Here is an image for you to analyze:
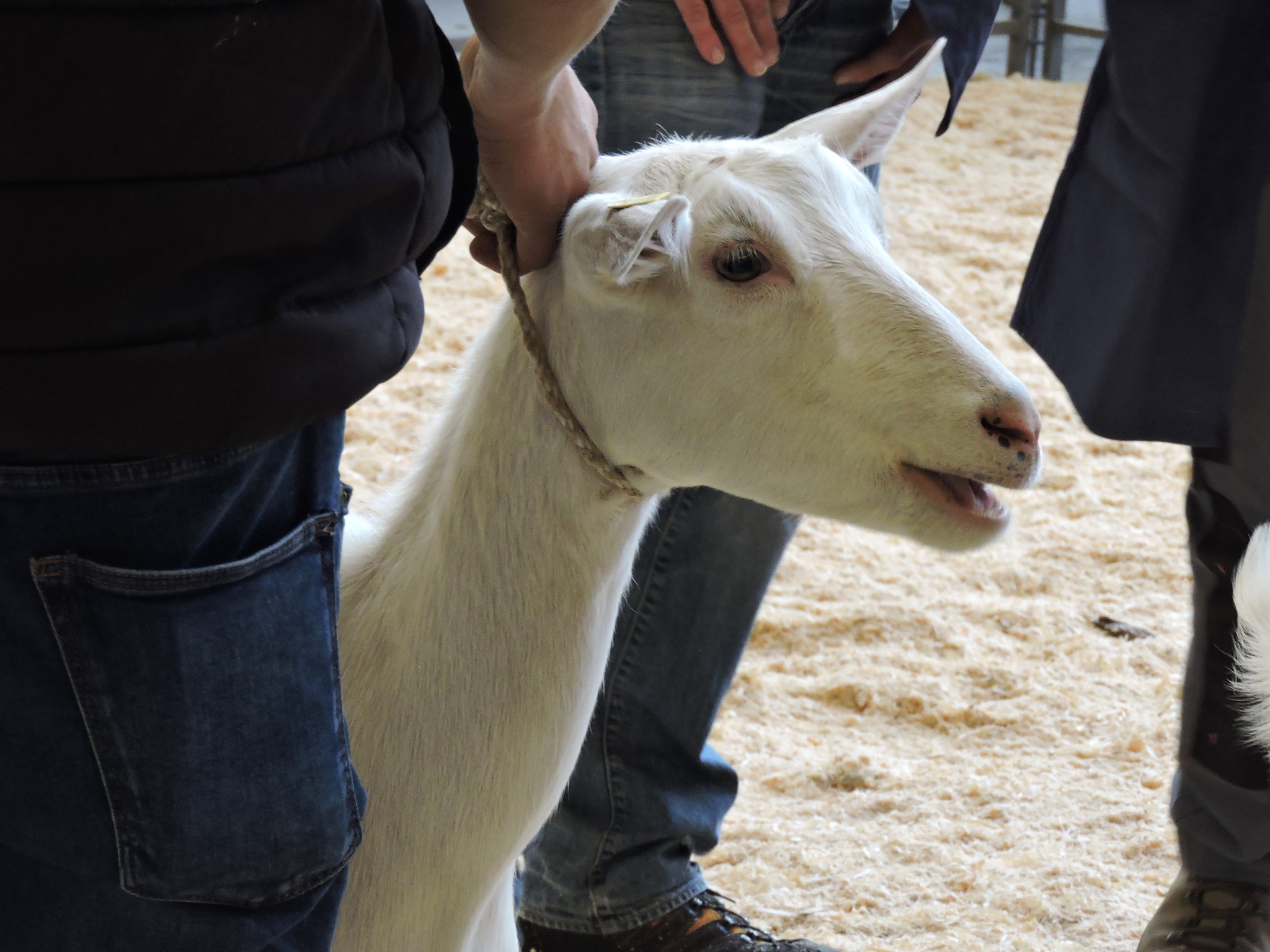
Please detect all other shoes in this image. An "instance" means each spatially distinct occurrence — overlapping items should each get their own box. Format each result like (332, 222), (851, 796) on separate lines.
(516, 889), (836, 952)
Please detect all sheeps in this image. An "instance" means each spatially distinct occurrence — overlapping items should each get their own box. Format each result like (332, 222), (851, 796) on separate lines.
(1227, 522), (1270, 756)
(325, 35), (1048, 952)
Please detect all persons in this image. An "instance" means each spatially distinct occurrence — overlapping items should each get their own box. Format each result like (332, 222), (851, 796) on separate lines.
(0, 0), (623, 952)
(501, 0), (1013, 952)
(1010, 0), (1270, 952)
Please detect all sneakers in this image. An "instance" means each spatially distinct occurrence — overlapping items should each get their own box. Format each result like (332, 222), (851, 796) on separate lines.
(1138, 869), (1269, 952)
